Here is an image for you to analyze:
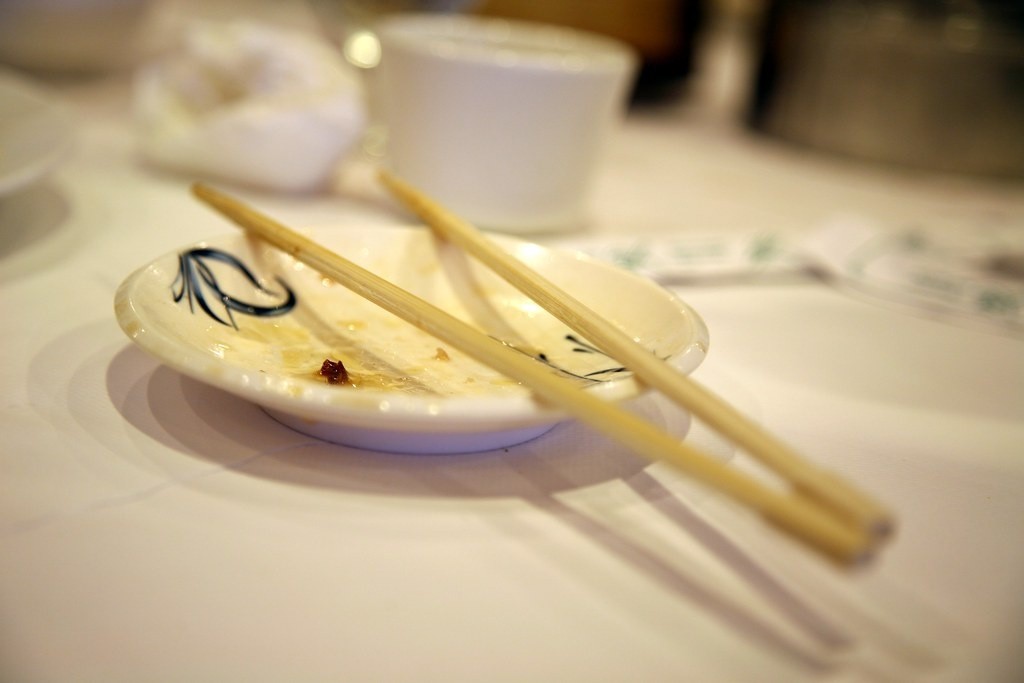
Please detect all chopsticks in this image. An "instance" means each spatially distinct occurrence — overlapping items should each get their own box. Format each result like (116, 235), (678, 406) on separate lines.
(193, 170), (893, 566)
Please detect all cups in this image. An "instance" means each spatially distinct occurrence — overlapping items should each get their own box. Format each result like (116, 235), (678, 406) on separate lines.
(384, 14), (632, 235)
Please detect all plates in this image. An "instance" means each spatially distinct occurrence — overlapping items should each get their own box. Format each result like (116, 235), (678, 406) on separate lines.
(0, 81), (77, 193)
(115, 228), (708, 453)
